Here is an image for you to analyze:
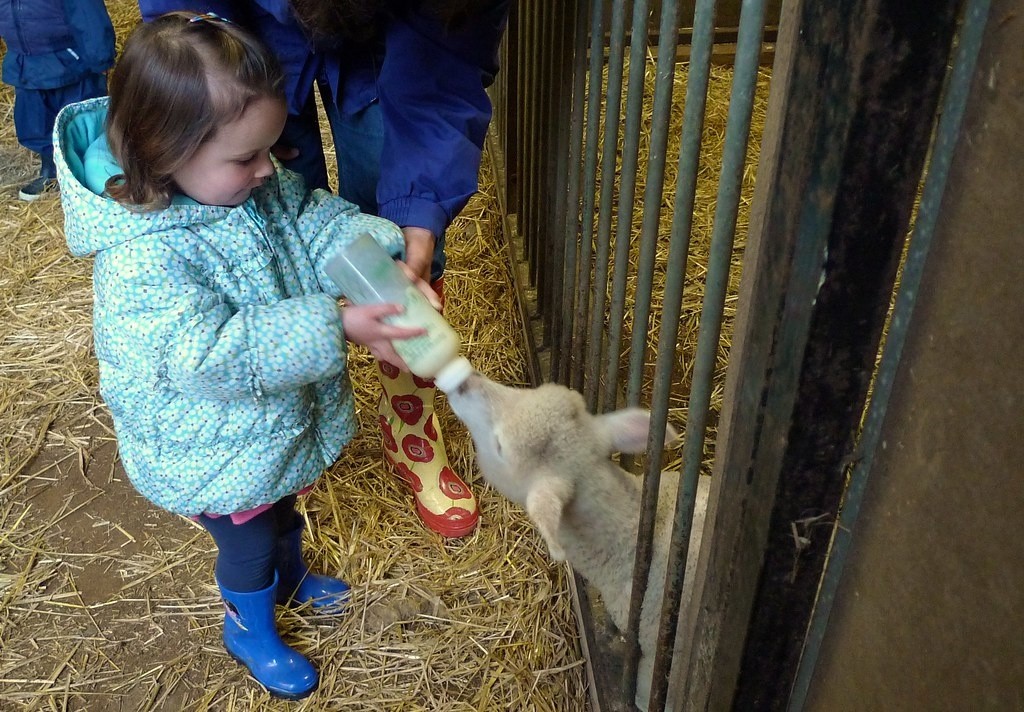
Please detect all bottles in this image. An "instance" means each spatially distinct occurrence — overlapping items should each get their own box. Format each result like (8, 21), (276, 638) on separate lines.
(322, 232), (473, 393)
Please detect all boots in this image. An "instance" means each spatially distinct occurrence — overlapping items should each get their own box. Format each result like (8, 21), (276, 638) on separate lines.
(373, 358), (480, 539)
(216, 564), (319, 699)
(269, 511), (351, 613)
(18, 145), (59, 200)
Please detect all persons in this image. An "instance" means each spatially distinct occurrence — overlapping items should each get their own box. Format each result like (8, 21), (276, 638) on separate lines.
(0, 0), (118, 202)
(137, 0), (512, 537)
(53, 9), (445, 700)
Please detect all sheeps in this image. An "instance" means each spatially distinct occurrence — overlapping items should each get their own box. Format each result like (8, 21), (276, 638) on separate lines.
(438, 372), (712, 712)
